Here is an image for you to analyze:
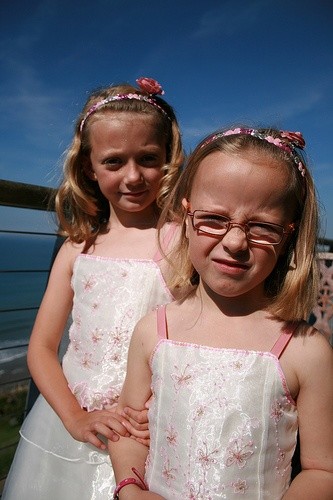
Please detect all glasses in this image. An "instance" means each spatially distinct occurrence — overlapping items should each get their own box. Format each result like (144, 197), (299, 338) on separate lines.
(186, 209), (297, 246)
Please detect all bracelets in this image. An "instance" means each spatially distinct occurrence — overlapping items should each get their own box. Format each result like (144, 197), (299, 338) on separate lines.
(112, 465), (150, 499)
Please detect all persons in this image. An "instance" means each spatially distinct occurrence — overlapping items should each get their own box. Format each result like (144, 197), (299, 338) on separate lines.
(106, 127), (333, 500)
(0, 76), (185, 500)
